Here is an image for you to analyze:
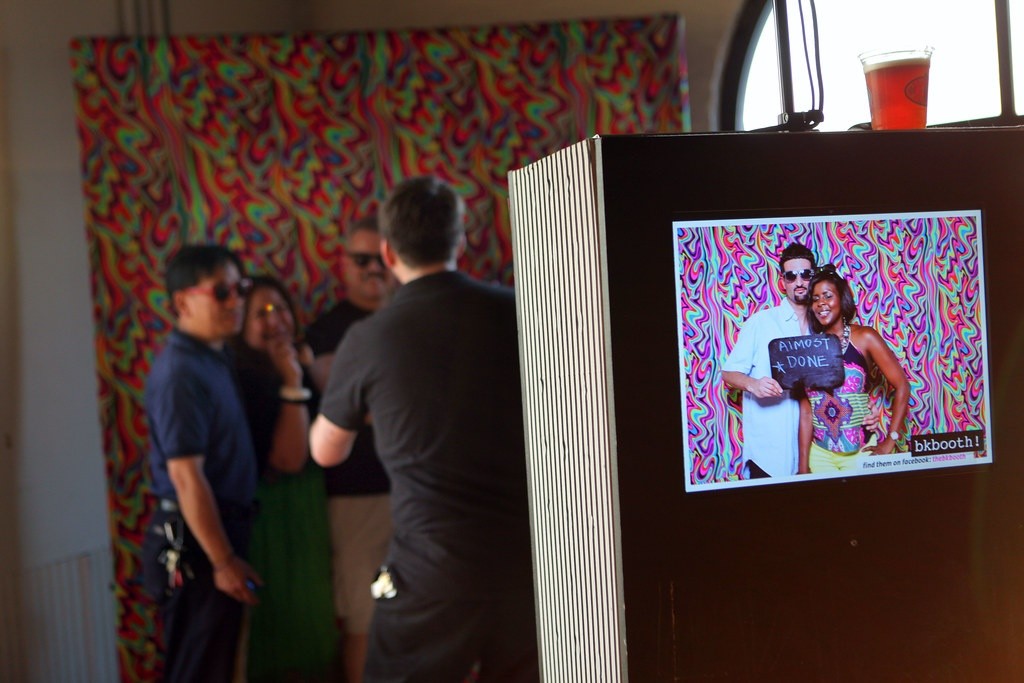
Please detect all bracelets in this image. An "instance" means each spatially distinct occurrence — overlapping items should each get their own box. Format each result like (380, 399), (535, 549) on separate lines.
(279, 387), (312, 400)
(216, 554), (236, 571)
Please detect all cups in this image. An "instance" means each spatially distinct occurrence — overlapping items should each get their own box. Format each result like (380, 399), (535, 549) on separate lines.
(859, 46), (932, 129)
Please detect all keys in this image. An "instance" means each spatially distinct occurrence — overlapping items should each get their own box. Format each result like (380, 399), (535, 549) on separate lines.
(165, 551), (182, 586)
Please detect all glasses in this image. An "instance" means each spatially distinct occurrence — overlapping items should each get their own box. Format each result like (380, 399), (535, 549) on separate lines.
(344, 250), (385, 270)
(185, 281), (243, 303)
(814, 264), (835, 275)
(781, 269), (814, 282)
(252, 303), (288, 316)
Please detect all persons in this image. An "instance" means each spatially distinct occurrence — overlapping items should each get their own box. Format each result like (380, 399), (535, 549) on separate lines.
(299, 204), (395, 683)
(797, 264), (911, 474)
(722, 244), (816, 479)
(307, 177), (538, 683)
(137, 244), (266, 683)
(229, 275), (343, 683)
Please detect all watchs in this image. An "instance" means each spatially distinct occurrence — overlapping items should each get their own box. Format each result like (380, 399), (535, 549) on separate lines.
(889, 431), (901, 441)
